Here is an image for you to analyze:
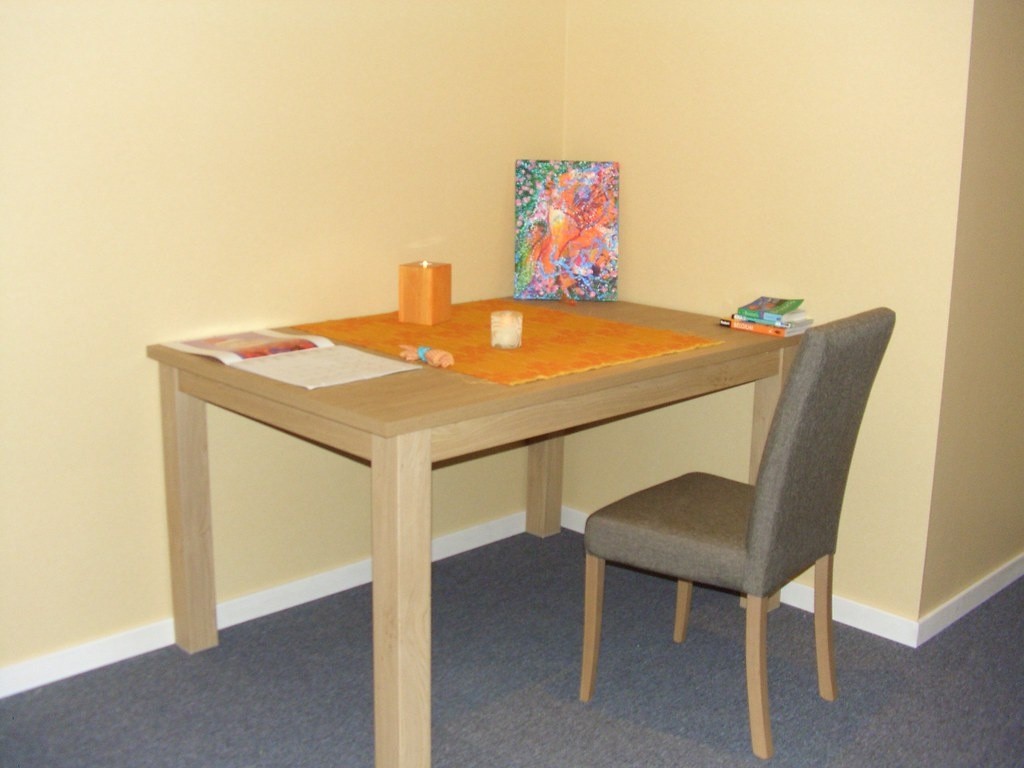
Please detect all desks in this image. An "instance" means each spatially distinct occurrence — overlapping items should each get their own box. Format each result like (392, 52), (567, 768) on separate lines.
(143, 294), (813, 768)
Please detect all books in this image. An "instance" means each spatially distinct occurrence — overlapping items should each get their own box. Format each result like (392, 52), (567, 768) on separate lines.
(720, 296), (814, 338)
(160, 329), (423, 390)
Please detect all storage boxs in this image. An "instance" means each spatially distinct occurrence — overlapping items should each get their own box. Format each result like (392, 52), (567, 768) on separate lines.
(397, 260), (452, 325)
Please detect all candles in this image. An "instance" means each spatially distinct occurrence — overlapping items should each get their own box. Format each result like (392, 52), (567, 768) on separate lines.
(489, 310), (523, 349)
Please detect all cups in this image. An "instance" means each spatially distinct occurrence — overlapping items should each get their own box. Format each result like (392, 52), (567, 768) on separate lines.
(491, 310), (523, 349)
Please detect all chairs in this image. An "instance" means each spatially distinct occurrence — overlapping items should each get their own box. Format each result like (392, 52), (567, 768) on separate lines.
(572, 298), (899, 760)
